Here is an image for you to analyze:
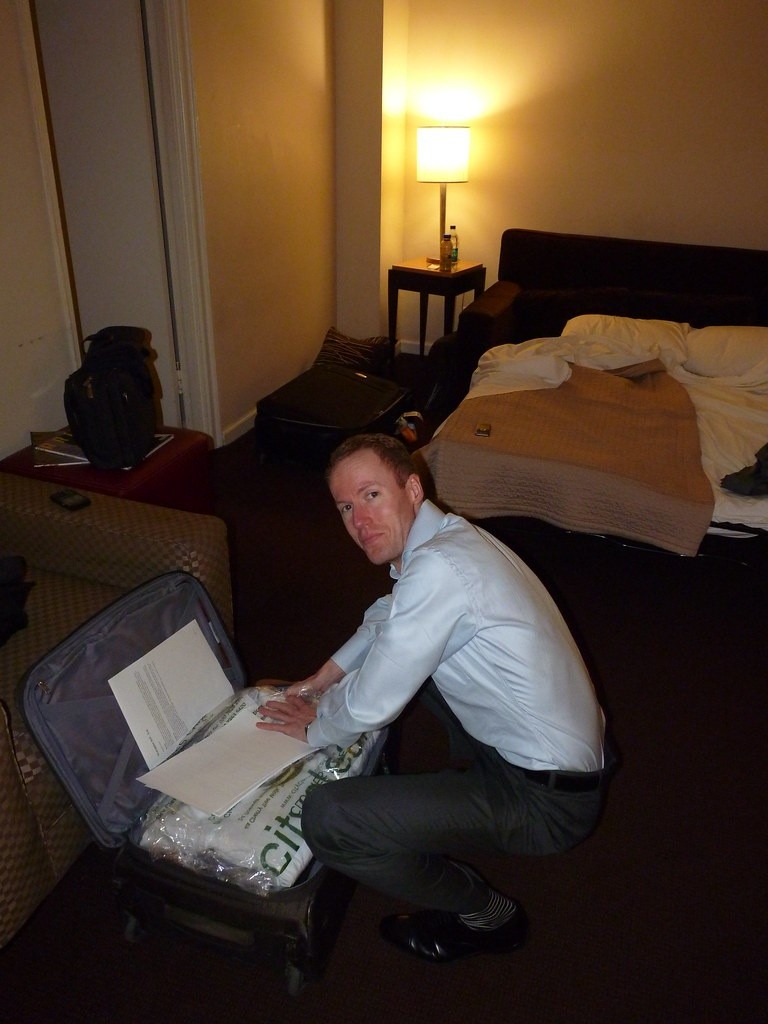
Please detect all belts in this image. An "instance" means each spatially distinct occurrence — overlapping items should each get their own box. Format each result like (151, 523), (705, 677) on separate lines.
(510, 765), (598, 793)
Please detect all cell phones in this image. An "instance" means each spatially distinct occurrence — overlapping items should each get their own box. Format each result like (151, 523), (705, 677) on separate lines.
(50, 489), (91, 511)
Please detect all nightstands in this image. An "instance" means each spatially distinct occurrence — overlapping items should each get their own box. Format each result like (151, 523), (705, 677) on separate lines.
(388, 267), (486, 355)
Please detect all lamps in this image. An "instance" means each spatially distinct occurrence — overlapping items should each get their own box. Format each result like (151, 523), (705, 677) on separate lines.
(392, 125), (484, 276)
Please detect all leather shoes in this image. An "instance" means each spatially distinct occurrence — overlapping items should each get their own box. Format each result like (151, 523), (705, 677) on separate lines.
(378, 900), (531, 963)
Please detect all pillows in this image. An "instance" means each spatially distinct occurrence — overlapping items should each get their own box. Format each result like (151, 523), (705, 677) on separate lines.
(562, 314), (689, 366)
(683, 323), (768, 375)
(311, 326), (389, 376)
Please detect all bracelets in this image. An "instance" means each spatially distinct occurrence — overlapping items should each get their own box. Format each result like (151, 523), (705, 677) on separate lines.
(304, 720), (315, 741)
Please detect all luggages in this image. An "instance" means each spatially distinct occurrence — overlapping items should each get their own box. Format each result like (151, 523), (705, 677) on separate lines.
(17, 571), (403, 970)
(256, 363), (414, 468)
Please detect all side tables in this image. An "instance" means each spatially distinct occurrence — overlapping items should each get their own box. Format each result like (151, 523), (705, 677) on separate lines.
(0, 425), (216, 512)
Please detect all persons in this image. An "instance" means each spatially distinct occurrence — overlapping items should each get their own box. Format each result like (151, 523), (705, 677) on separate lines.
(257, 430), (612, 962)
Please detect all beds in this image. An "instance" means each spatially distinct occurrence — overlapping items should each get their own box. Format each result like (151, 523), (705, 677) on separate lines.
(438, 229), (768, 589)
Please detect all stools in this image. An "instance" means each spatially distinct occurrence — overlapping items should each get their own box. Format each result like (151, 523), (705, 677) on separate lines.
(252, 364), (414, 482)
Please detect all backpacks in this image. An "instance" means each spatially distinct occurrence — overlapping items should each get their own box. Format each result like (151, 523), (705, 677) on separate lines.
(63, 326), (159, 468)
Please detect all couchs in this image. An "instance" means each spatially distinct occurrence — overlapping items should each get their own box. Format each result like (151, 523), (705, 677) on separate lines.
(0, 467), (235, 951)
